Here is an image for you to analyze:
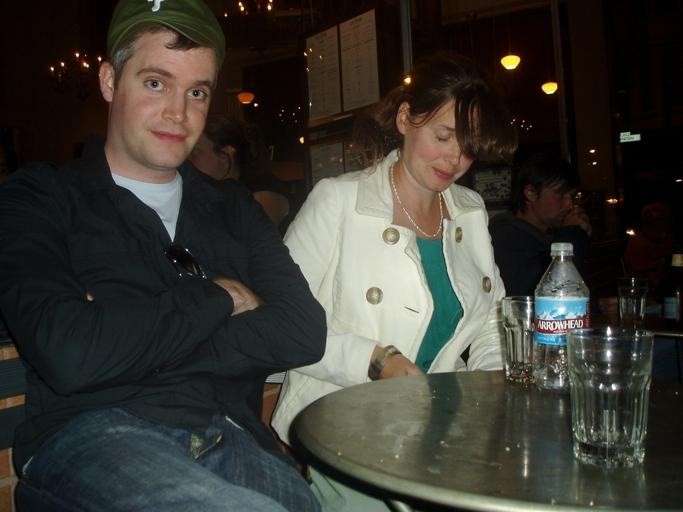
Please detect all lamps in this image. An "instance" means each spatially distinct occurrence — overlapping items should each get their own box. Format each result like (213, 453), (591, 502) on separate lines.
(541, 81), (559, 96)
(239, 93), (255, 105)
(501, 54), (520, 71)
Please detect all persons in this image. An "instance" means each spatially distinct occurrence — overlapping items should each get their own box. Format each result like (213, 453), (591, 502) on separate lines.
(491, 145), (592, 300)
(0, 0), (327, 512)
(188, 117), (295, 239)
(270, 66), (514, 511)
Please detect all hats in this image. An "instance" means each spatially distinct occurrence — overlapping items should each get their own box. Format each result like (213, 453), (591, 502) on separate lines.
(107, 0), (227, 69)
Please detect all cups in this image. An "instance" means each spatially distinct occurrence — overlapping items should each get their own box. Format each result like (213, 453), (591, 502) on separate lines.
(618, 277), (648, 327)
(501, 295), (536, 384)
(567, 328), (654, 468)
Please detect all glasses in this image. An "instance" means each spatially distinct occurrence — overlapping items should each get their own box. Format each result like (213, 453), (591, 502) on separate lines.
(163, 242), (207, 280)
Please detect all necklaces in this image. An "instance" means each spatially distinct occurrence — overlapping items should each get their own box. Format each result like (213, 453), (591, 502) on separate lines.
(390, 162), (445, 237)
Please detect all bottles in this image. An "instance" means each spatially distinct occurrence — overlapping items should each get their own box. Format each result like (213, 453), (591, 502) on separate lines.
(532, 243), (590, 392)
(624, 276), (639, 315)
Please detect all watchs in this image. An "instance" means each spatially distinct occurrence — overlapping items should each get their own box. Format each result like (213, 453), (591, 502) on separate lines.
(368, 343), (401, 381)
